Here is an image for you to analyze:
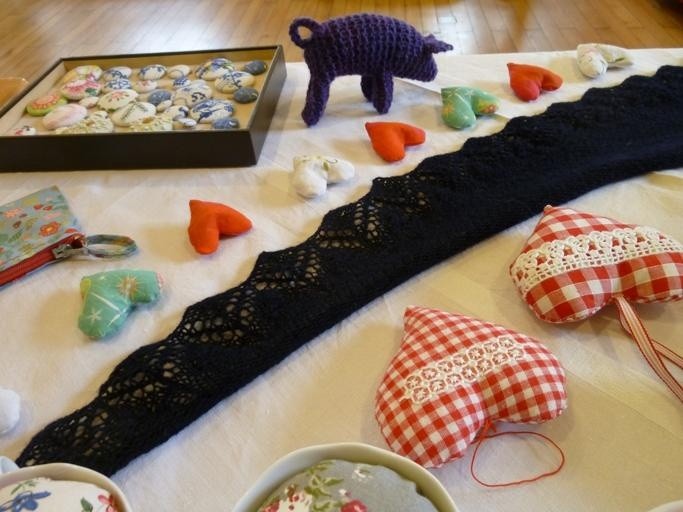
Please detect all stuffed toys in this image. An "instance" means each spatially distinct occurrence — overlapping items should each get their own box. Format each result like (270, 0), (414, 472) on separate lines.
(75, 266), (165, 344)
(440, 85), (499, 131)
(364, 120), (428, 164)
(187, 198), (254, 255)
(507, 61), (564, 102)
(372, 304), (571, 471)
(288, 12), (454, 129)
(292, 153), (356, 199)
(508, 204), (683, 326)
(577, 42), (634, 79)
